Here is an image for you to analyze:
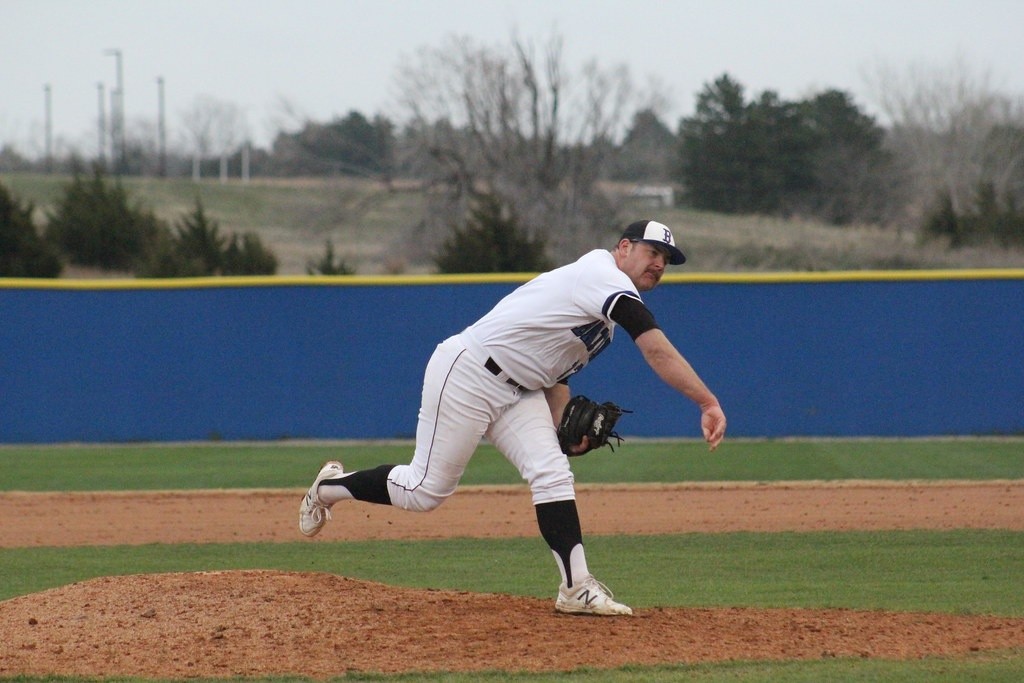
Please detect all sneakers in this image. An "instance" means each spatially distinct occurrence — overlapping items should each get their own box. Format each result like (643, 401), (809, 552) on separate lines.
(554, 574), (633, 615)
(298, 461), (344, 537)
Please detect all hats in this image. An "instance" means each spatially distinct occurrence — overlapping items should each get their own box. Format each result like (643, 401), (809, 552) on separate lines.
(619, 220), (686, 265)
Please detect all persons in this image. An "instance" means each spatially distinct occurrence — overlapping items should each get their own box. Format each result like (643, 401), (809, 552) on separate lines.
(299, 220), (727, 617)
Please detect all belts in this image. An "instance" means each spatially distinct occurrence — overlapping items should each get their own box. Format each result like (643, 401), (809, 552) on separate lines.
(484, 356), (526, 393)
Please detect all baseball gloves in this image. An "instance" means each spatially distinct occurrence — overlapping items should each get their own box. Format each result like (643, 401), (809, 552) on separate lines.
(556, 394), (634, 458)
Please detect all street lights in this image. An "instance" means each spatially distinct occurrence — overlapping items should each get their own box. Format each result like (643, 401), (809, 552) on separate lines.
(105, 49), (125, 160)
